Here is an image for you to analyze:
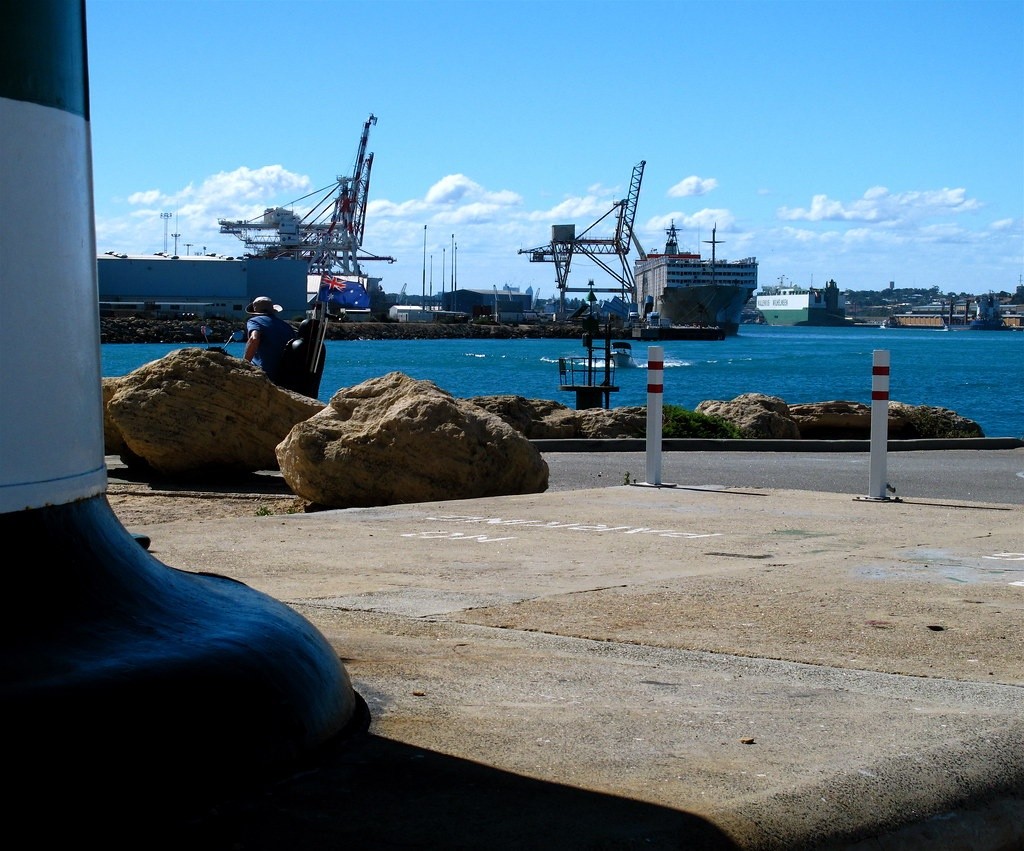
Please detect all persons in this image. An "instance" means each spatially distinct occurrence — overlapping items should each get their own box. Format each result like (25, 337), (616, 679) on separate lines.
(242, 297), (296, 390)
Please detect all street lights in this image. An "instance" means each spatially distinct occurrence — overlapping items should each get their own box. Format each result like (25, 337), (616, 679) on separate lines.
(183, 244), (194, 256)
(422, 224), (458, 297)
(160, 212), (173, 254)
(171, 233), (181, 255)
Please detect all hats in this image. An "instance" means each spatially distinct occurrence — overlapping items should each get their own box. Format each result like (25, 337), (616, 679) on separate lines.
(245, 296), (283, 316)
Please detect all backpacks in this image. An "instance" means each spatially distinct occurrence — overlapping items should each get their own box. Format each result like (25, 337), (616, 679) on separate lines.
(283, 318), (327, 398)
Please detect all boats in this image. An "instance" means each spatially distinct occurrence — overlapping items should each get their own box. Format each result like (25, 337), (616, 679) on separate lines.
(755, 277), (855, 327)
(609, 342), (634, 368)
(632, 217), (760, 338)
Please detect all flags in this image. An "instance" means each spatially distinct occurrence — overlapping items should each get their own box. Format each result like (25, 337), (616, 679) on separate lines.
(315, 273), (371, 308)
